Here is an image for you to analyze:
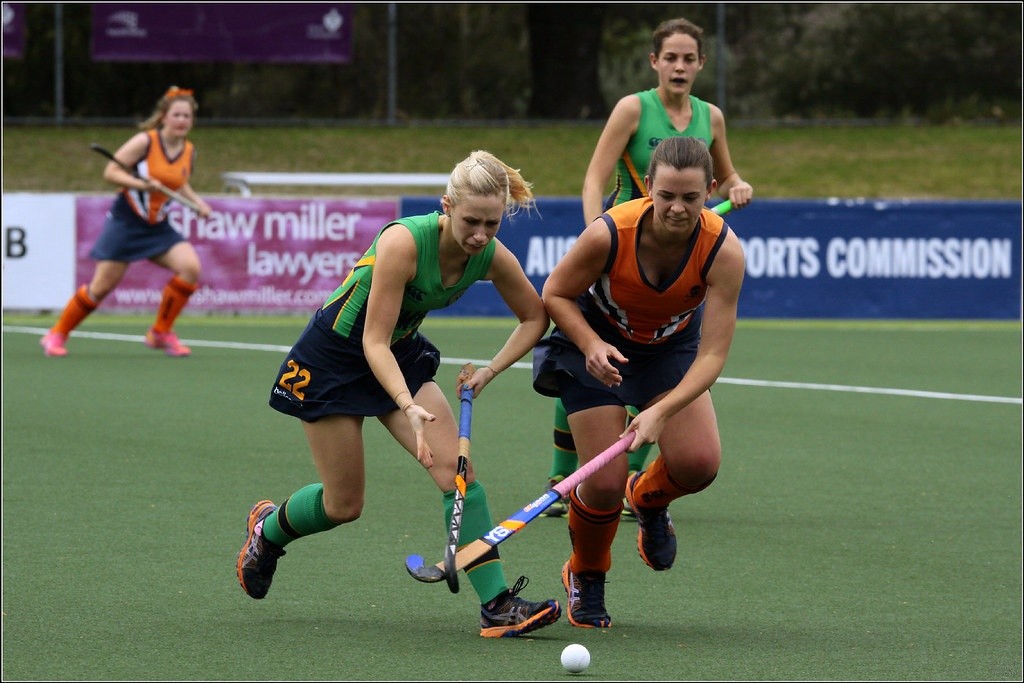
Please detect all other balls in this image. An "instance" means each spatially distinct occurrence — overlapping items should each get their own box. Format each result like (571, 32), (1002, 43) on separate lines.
(559, 644), (591, 673)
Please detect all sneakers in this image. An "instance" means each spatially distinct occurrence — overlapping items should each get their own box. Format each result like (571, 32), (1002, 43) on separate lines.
(236, 500), (286, 600)
(480, 575), (562, 638)
(621, 469), (642, 518)
(625, 471), (677, 571)
(40, 336), (67, 358)
(538, 475), (570, 518)
(561, 560), (612, 628)
(145, 328), (190, 357)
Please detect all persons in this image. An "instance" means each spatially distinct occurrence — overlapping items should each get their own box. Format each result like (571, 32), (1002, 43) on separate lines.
(538, 17), (754, 518)
(237, 147), (562, 641)
(530, 137), (746, 631)
(39, 87), (218, 357)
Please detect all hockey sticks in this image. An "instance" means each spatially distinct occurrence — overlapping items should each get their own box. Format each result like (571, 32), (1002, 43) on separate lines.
(443, 362), (474, 595)
(707, 200), (739, 219)
(403, 429), (639, 585)
(87, 142), (205, 214)
